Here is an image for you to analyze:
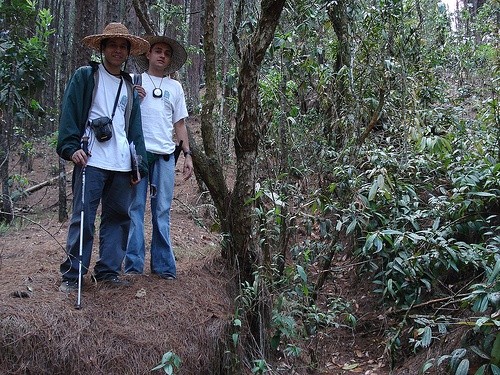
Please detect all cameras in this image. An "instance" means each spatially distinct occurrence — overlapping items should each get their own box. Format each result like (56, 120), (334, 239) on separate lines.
(91, 116), (112, 142)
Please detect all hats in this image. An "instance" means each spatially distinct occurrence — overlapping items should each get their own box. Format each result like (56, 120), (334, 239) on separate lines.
(82, 23), (150, 56)
(132, 35), (188, 76)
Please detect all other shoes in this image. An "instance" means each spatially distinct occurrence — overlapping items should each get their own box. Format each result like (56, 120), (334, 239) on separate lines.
(124, 270), (140, 277)
(158, 272), (176, 281)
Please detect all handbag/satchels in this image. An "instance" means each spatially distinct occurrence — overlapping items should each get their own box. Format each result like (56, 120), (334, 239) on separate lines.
(90, 115), (114, 142)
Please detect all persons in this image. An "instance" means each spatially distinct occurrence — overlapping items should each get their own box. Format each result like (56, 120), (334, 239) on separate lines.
(123, 34), (194, 279)
(56, 22), (149, 293)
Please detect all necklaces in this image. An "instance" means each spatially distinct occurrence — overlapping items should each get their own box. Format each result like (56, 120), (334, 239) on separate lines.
(147, 73), (163, 97)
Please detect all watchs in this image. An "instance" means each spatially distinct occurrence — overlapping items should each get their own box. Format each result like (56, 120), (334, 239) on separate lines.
(183, 150), (192, 157)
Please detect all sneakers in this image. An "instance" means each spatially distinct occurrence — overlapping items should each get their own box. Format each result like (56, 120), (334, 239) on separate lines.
(92, 276), (132, 290)
(59, 279), (86, 293)
